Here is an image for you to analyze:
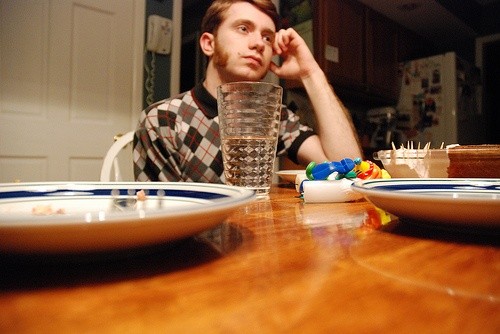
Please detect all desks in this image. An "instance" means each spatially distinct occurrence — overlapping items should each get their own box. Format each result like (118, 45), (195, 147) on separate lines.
(0, 183), (500, 334)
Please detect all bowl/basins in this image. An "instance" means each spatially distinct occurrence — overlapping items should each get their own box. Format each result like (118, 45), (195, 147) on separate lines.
(446, 145), (500, 178)
(275, 170), (308, 183)
(378, 150), (450, 178)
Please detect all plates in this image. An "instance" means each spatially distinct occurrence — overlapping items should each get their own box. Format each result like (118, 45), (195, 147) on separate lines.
(0, 182), (256, 253)
(351, 178), (500, 222)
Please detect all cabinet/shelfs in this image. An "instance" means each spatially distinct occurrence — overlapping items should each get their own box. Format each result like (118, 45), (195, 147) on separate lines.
(279, 0), (431, 100)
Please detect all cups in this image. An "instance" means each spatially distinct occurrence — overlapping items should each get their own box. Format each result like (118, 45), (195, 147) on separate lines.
(217, 82), (283, 199)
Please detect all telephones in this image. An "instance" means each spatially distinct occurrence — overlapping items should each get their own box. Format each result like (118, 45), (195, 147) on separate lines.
(147, 14), (173, 54)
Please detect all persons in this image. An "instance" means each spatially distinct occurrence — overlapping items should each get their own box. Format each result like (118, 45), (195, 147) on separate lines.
(132, 0), (363, 184)
